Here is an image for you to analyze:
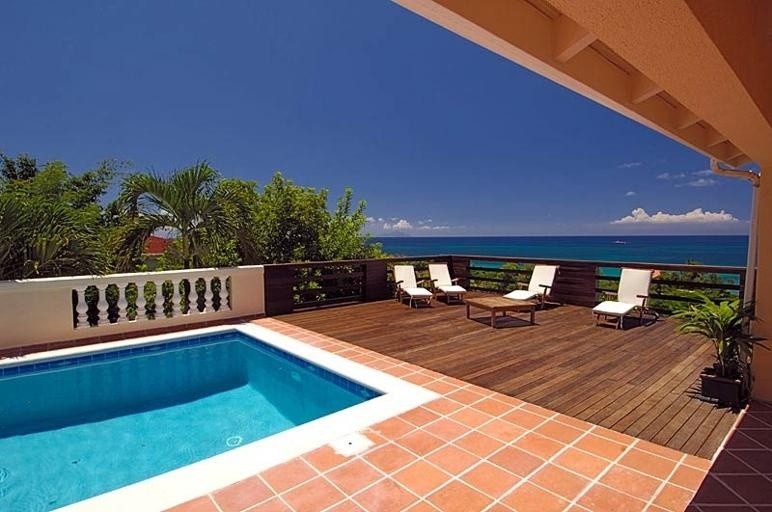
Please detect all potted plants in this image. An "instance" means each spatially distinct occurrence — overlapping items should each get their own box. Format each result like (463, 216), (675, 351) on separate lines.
(667, 290), (768, 403)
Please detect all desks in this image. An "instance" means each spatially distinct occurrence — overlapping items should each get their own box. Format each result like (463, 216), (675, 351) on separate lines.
(463, 296), (537, 328)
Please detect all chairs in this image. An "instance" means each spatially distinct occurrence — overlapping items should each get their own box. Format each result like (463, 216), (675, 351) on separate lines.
(428, 262), (467, 306)
(394, 265), (434, 309)
(591, 266), (660, 330)
(502, 264), (564, 309)
